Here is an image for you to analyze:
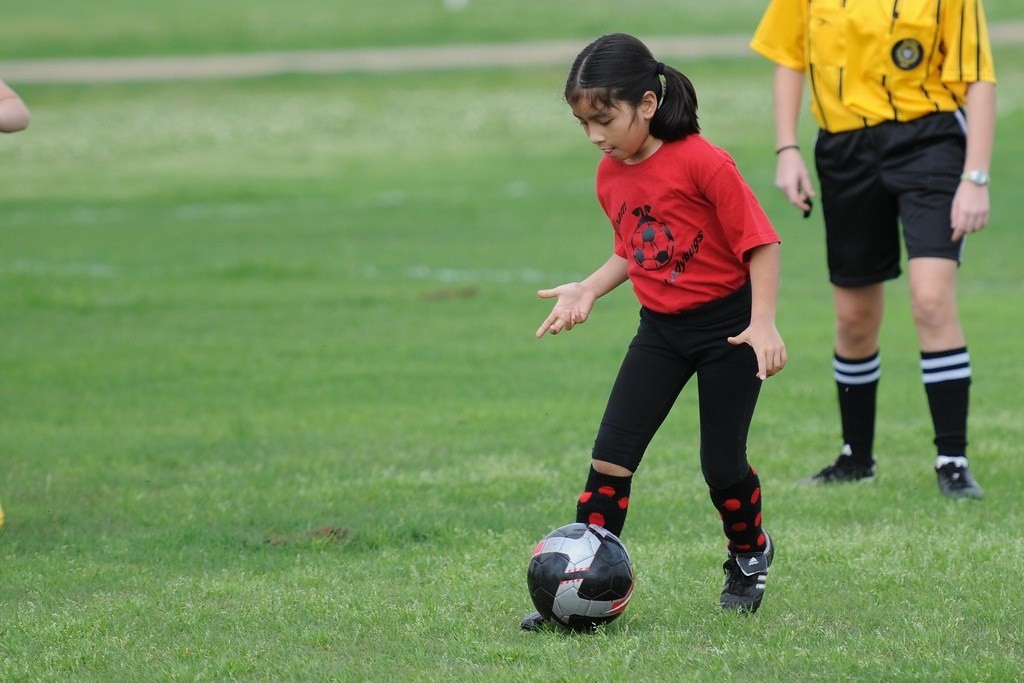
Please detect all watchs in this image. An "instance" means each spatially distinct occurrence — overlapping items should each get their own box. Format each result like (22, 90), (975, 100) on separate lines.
(960, 170), (990, 186)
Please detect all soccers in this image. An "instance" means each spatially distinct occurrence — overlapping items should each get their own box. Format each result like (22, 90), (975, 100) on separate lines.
(527, 524), (634, 631)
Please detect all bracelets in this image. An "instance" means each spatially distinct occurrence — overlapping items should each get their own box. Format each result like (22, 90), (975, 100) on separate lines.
(776, 145), (799, 154)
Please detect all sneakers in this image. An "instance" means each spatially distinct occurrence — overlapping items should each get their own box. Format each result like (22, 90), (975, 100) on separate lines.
(520, 611), (568, 633)
(933, 456), (985, 502)
(795, 454), (877, 488)
(721, 527), (773, 616)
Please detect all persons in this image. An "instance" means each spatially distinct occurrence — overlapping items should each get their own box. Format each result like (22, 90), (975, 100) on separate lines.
(0, 79), (30, 133)
(520, 33), (788, 635)
(748, 0), (998, 502)
